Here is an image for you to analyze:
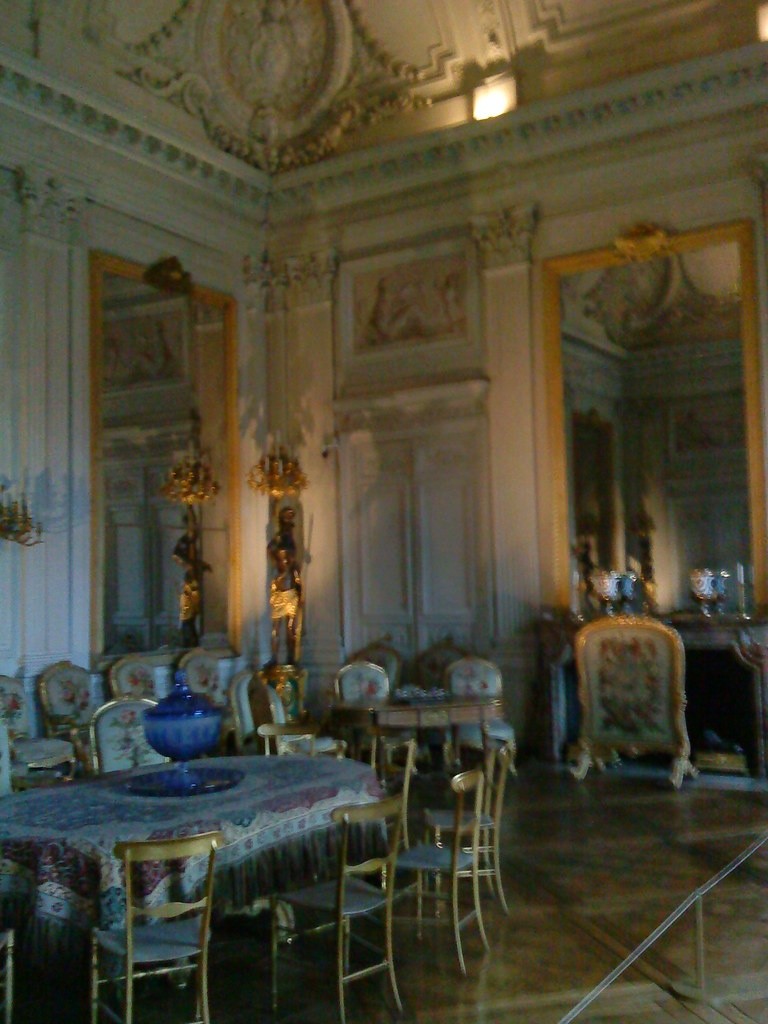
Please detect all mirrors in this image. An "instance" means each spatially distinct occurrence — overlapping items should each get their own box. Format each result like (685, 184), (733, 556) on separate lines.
(540, 214), (764, 626)
(86, 249), (243, 667)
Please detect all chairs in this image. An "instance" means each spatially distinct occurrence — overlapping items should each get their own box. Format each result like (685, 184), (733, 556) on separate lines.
(0, 646), (511, 1024)
(565, 612), (703, 791)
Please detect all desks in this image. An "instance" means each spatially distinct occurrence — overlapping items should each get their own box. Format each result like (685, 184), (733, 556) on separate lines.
(329, 697), (506, 778)
(0, 749), (385, 1001)
